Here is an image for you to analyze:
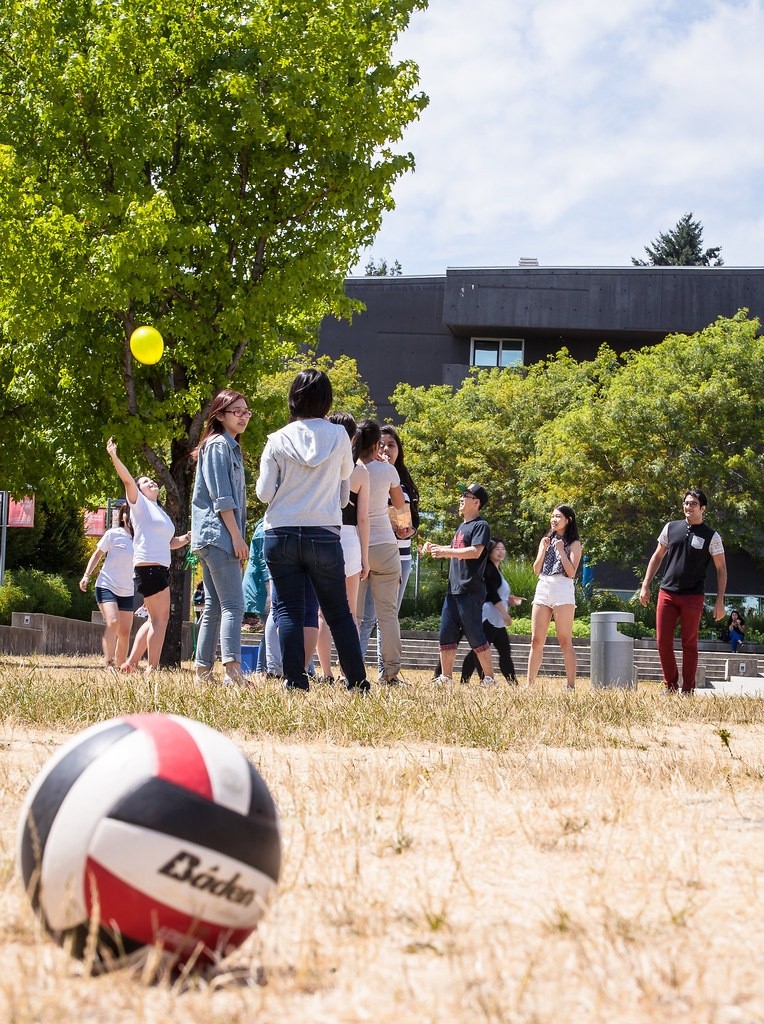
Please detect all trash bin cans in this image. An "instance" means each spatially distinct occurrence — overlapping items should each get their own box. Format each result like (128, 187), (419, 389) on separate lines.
(590, 609), (636, 692)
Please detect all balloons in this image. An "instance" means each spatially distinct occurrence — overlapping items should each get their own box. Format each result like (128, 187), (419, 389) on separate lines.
(129, 325), (163, 365)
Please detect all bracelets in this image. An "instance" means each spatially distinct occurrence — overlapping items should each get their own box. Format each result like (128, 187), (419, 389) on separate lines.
(561, 555), (567, 562)
(84, 572), (93, 579)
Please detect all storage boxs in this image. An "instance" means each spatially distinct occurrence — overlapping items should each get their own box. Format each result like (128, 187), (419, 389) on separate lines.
(240, 644), (259, 672)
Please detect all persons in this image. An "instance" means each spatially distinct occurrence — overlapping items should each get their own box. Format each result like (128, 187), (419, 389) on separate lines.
(639, 489), (728, 697)
(431, 536), (528, 688)
(525, 505), (583, 694)
(187, 387), (258, 691)
(727, 610), (745, 653)
(255, 367), (371, 693)
(422, 482), (496, 689)
(241, 411), (421, 689)
(79, 503), (135, 674)
(105, 436), (192, 678)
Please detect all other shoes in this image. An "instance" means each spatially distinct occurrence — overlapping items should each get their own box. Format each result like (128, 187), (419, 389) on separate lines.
(377, 673), (411, 688)
(105, 658), (120, 673)
(194, 674), (223, 686)
(318, 675), (334, 685)
(739, 641), (744, 647)
(144, 669), (162, 678)
(120, 662), (141, 675)
(481, 675), (496, 688)
(431, 674), (453, 686)
(222, 674), (257, 689)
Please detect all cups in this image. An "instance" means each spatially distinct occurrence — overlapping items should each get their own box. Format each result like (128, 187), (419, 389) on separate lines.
(712, 633), (717, 641)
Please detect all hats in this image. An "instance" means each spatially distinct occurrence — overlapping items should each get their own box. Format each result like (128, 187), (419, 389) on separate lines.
(458, 482), (488, 505)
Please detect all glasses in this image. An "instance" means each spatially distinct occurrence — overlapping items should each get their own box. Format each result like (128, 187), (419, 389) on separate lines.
(682, 501), (700, 508)
(460, 493), (476, 499)
(222, 409), (252, 418)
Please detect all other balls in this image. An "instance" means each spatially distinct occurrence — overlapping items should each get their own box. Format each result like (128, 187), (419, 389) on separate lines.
(9, 710), (285, 985)
